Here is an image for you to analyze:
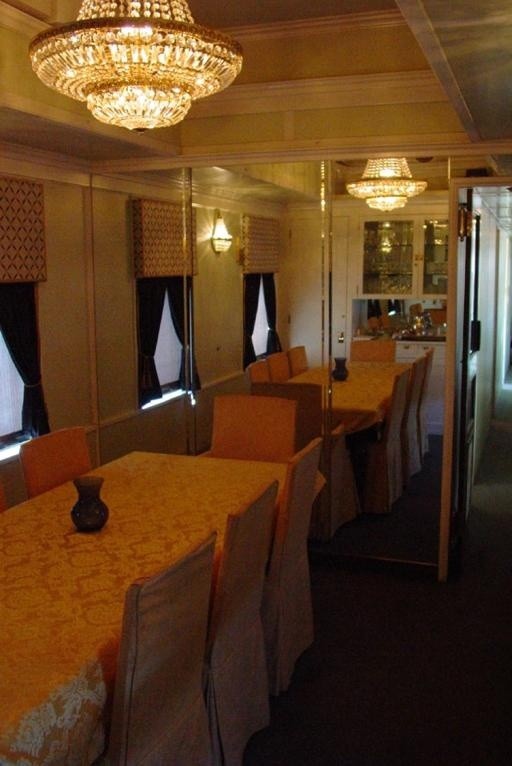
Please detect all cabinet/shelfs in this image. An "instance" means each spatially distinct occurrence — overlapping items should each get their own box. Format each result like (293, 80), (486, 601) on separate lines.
(347, 208), (452, 301)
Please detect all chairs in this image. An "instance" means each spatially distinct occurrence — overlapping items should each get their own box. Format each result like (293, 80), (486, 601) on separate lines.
(247, 358), (269, 395)
(199, 394), (296, 465)
(353, 335), (445, 437)
(94, 528), (211, 766)
(349, 339), (396, 362)
(252, 382), (343, 539)
(349, 367), (409, 514)
(398, 357), (424, 483)
(290, 345), (309, 379)
(267, 351), (290, 384)
(260, 437), (320, 694)
(204, 479), (277, 764)
(19, 426), (90, 500)
(418, 349), (434, 468)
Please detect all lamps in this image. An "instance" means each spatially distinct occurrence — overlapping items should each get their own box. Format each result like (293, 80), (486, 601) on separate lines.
(345, 159), (429, 211)
(212, 209), (233, 252)
(28, 1), (245, 134)
